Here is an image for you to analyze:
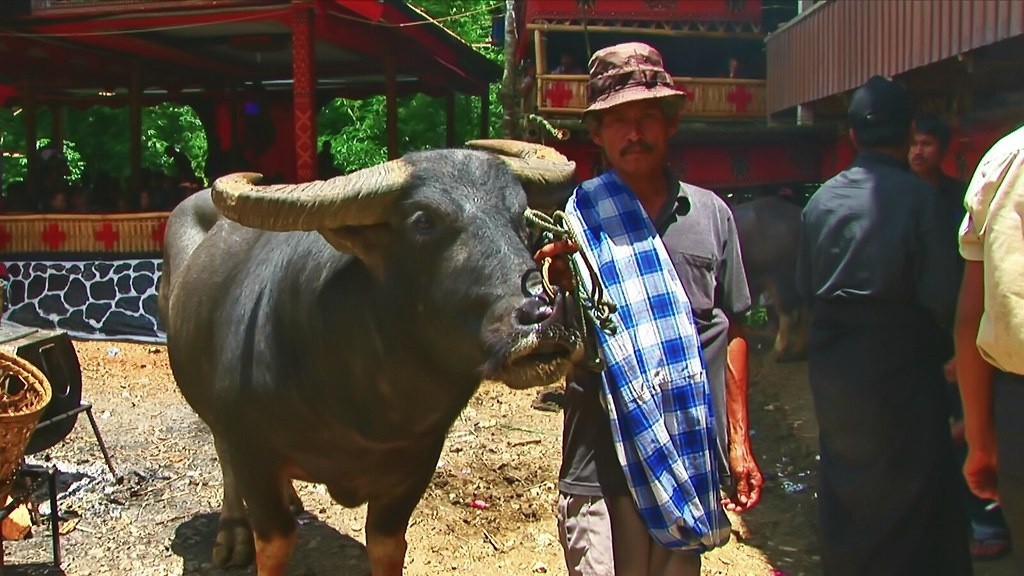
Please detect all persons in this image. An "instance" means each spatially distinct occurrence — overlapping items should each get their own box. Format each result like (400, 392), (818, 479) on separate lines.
(954, 126), (1024, 564)
(795, 77), (968, 576)
(906, 117), (1011, 559)
(533, 42), (762, 576)
(22, 135), (348, 213)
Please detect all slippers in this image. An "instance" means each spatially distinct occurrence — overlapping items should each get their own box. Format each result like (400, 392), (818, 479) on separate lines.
(970, 537), (1008, 559)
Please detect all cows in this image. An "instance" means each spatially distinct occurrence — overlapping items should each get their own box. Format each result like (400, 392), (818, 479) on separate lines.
(158, 139), (587, 576)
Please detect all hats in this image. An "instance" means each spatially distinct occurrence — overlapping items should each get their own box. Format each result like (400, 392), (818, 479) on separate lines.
(847, 75), (912, 132)
(581, 42), (687, 120)
